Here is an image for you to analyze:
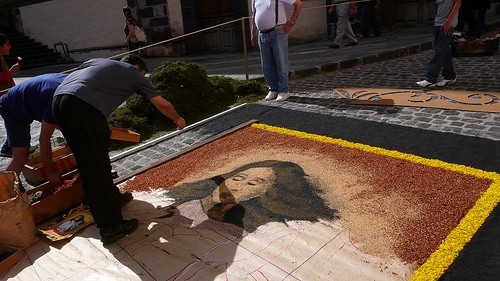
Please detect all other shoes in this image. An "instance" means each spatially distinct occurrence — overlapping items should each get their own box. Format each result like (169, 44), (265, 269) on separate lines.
(345, 42), (358, 46)
(1, 147), (12, 157)
(329, 44), (340, 48)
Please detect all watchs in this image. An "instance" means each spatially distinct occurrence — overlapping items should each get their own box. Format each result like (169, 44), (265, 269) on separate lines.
(289, 19), (296, 25)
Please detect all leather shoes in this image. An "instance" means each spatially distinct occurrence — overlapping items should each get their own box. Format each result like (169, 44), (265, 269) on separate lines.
(100, 219), (139, 245)
(120, 191), (133, 208)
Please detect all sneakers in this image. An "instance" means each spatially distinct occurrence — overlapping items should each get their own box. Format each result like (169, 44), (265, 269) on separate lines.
(436, 78), (456, 86)
(276, 90), (290, 102)
(265, 90), (278, 100)
(416, 80), (437, 88)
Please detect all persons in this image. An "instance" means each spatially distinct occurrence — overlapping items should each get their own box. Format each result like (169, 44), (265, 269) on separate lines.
(326, 0), (397, 49)
(0, 74), (70, 193)
(450, 0), (492, 42)
(416, 0), (461, 88)
(251, 0), (302, 101)
(123, 7), (147, 54)
(53, 54), (186, 247)
(0, 35), (38, 158)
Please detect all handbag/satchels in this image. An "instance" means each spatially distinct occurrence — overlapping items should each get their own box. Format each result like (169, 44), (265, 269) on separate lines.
(135, 26), (147, 42)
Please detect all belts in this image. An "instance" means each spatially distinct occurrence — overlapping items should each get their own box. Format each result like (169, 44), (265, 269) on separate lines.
(260, 28), (274, 34)
